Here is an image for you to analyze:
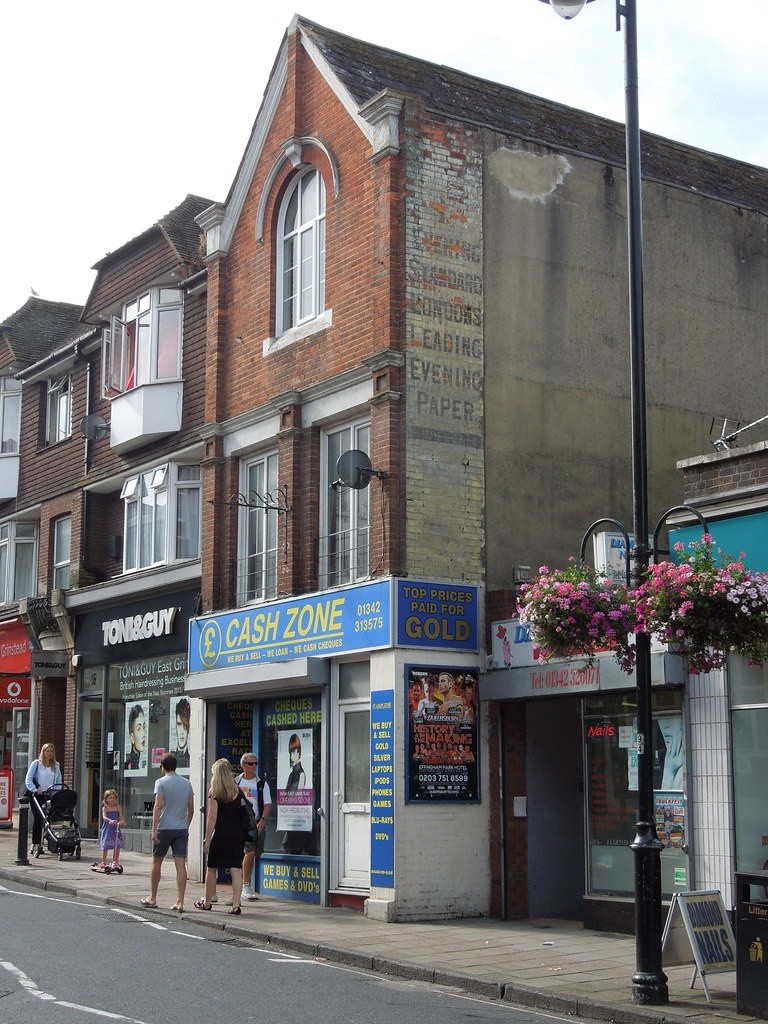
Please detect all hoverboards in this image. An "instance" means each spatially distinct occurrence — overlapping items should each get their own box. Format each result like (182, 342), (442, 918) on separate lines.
(90, 822), (123, 875)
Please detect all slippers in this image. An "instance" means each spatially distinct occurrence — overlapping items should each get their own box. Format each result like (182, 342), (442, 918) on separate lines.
(169, 905), (185, 913)
(141, 898), (158, 909)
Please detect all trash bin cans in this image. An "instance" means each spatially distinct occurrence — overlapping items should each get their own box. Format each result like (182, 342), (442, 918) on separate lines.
(733, 869), (768, 1018)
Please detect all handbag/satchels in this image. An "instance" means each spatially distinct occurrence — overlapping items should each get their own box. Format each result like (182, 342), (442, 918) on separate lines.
(17, 760), (41, 798)
(239, 797), (260, 843)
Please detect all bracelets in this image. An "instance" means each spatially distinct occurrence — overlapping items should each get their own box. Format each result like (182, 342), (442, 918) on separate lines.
(261, 818), (267, 822)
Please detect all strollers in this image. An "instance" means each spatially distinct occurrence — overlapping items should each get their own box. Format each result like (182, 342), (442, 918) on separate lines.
(30, 783), (82, 861)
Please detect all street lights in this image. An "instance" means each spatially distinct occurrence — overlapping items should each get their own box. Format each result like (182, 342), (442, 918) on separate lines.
(534, 0), (669, 1005)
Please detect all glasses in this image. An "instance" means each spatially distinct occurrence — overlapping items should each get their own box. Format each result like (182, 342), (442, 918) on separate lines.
(243, 762), (258, 766)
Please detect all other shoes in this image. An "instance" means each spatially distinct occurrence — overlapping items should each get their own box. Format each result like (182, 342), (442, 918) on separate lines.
(225, 895), (242, 906)
(29, 844), (36, 854)
(100, 862), (106, 868)
(241, 883), (257, 900)
(201, 894), (217, 902)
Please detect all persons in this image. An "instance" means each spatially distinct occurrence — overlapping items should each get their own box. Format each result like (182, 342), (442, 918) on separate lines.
(99, 788), (126, 866)
(232, 753), (272, 900)
(411, 672), (477, 727)
(169, 698), (191, 768)
(284, 734), (306, 791)
(125, 704), (146, 770)
(663, 809), (676, 823)
(139, 753), (194, 912)
(194, 757), (258, 915)
(657, 717), (684, 791)
(25, 742), (62, 855)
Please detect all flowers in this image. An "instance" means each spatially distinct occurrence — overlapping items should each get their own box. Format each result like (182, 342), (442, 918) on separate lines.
(512, 567), (638, 677)
(637, 533), (768, 680)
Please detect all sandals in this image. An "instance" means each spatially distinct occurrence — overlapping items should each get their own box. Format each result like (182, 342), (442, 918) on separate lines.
(227, 906), (241, 915)
(194, 900), (212, 910)
